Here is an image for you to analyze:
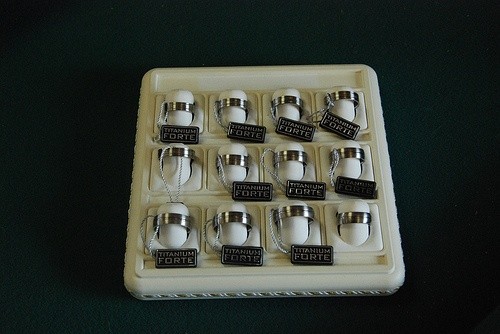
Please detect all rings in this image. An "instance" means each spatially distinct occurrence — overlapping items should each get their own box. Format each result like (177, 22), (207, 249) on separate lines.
(330, 147), (365, 165)
(215, 97), (249, 115)
(215, 154), (249, 168)
(273, 205), (316, 225)
(272, 149), (308, 167)
(323, 89), (360, 108)
(163, 101), (196, 115)
(158, 146), (196, 161)
(212, 211), (253, 231)
(152, 212), (192, 233)
(336, 211), (372, 225)
(269, 94), (306, 119)
